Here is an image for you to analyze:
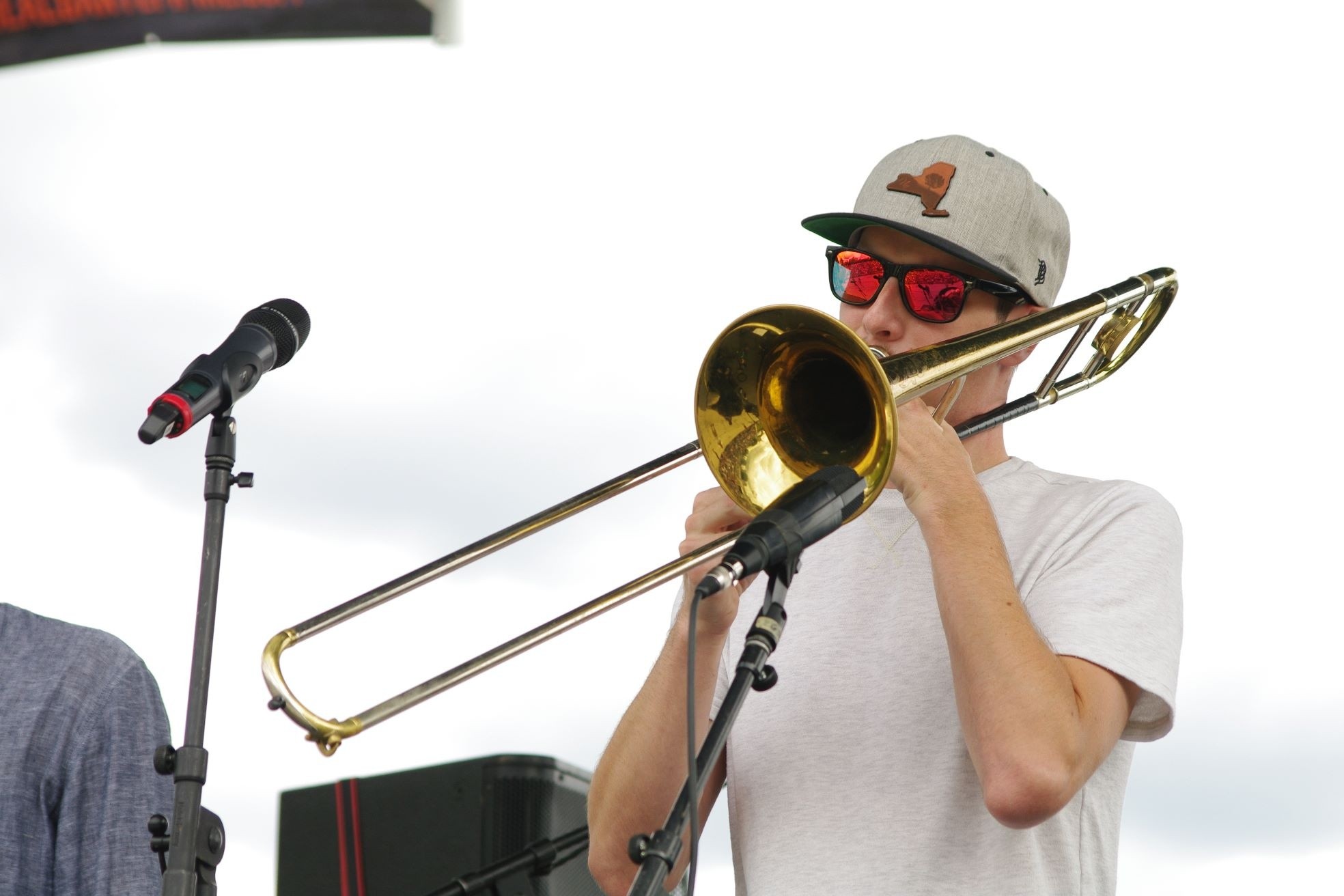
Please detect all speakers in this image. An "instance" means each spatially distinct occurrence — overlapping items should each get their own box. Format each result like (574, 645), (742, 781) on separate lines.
(275, 755), (606, 896)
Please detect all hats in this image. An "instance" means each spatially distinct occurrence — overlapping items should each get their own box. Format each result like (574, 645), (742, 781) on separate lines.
(801, 135), (1070, 308)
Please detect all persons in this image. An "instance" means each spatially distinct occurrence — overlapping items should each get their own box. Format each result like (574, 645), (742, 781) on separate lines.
(0, 605), (172, 896)
(584, 135), (1183, 896)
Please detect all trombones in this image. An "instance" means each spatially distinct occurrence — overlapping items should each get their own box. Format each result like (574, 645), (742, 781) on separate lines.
(259, 266), (1179, 756)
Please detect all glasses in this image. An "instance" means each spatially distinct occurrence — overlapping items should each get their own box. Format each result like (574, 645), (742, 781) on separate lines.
(825, 244), (1035, 324)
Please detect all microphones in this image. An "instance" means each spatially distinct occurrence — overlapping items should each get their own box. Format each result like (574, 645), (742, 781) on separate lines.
(694, 465), (865, 599)
(138, 299), (311, 446)
